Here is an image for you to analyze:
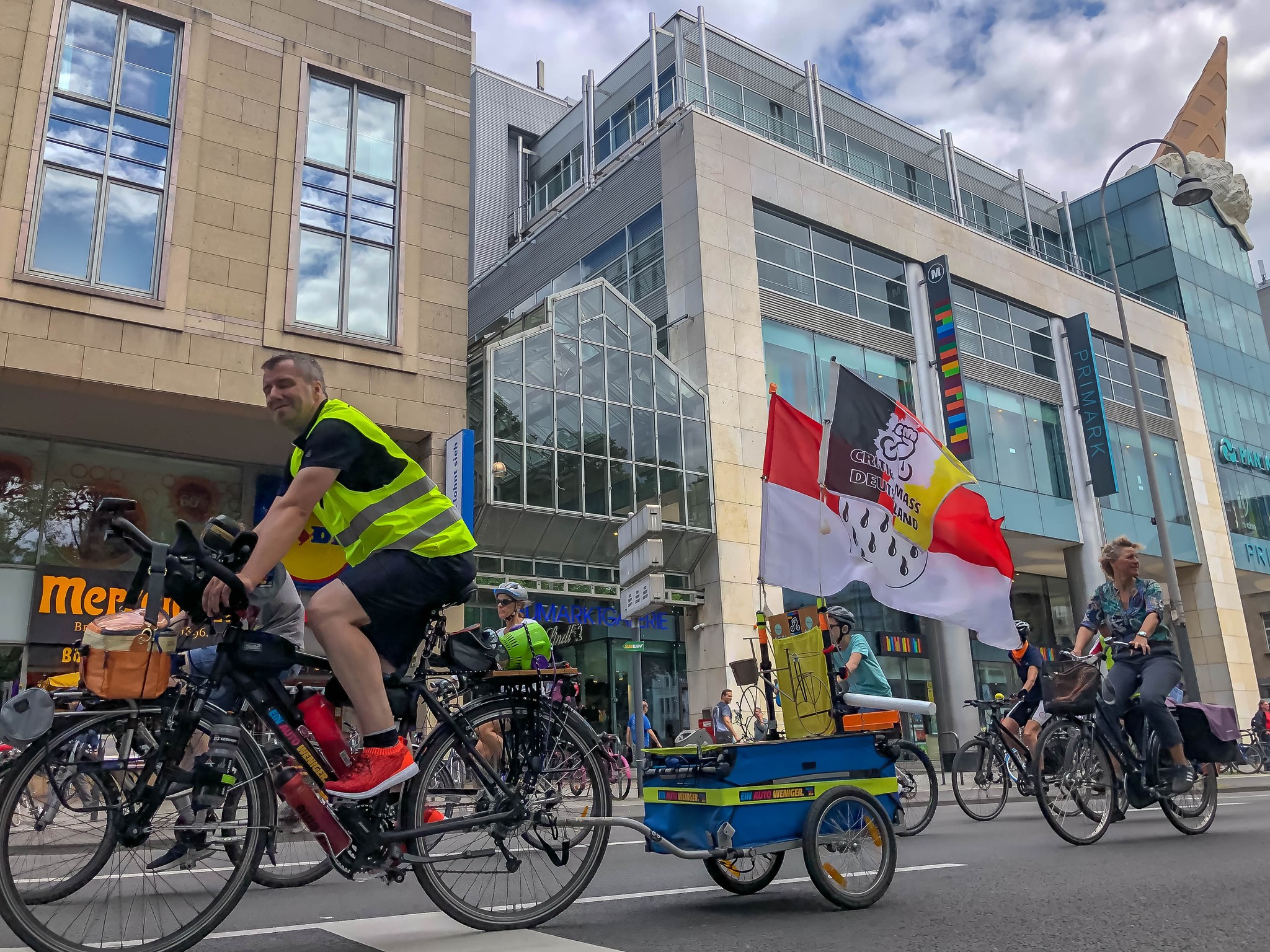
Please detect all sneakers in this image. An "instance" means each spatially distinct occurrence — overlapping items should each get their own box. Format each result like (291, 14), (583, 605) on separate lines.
(1092, 777), (1124, 792)
(1172, 759), (1196, 794)
(388, 805), (451, 872)
(322, 735), (420, 800)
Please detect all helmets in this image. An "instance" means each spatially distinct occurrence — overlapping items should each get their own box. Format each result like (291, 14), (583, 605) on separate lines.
(493, 581), (529, 610)
(826, 605), (857, 630)
(1013, 619), (1030, 635)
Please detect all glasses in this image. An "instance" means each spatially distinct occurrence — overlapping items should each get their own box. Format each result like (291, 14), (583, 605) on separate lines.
(495, 598), (515, 607)
(830, 624), (840, 630)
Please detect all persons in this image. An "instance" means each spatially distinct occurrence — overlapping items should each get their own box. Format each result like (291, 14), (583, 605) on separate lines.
(827, 605), (892, 714)
(1250, 700), (1270, 771)
(1069, 538), (1198, 793)
(1000, 620), (1056, 795)
(713, 689), (740, 744)
(202, 354), (478, 874)
(754, 707), (768, 742)
(469, 582), (539, 829)
(627, 701), (662, 758)
(146, 515), (304, 872)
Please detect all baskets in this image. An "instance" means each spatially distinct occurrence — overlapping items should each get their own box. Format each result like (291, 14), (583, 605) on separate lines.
(1038, 660), (1101, 718)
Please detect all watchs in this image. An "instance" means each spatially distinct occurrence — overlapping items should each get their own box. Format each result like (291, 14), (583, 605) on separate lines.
(1136, 631), (1148, 639)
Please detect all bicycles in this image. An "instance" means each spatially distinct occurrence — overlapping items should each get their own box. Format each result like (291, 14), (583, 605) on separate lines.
(1032, 635), (1219, 846)
(834, 692), (939, 837)
(982, 713), (1270, 786)
(952, 692), (1092, 821)
(0, 495), (631, 952)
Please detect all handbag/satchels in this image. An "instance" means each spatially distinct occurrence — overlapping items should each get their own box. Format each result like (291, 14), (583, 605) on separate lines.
(68, 603), (180, 701)
(1175, 702), (1241, 763)
(1047, 666), (1099, 707)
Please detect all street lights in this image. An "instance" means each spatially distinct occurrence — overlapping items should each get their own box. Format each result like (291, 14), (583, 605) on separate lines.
(1099, 138), (1212, 704)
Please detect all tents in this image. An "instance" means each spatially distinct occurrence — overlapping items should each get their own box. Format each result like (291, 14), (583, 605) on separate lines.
(41, 672), (80, 690)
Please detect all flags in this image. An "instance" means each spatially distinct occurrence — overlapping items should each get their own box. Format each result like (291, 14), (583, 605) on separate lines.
(758, 356), (1023, 650)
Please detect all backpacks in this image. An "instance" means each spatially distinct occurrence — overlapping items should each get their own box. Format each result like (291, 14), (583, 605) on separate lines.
(1250, 716), (1258, 733)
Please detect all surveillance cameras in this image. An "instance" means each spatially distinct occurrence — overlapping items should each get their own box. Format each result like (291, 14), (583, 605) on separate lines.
(693, 625), (700, 631)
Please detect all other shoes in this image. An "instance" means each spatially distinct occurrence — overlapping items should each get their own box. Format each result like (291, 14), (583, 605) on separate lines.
(145, 831), (217, 873)
(166, 773), (195, 801)
